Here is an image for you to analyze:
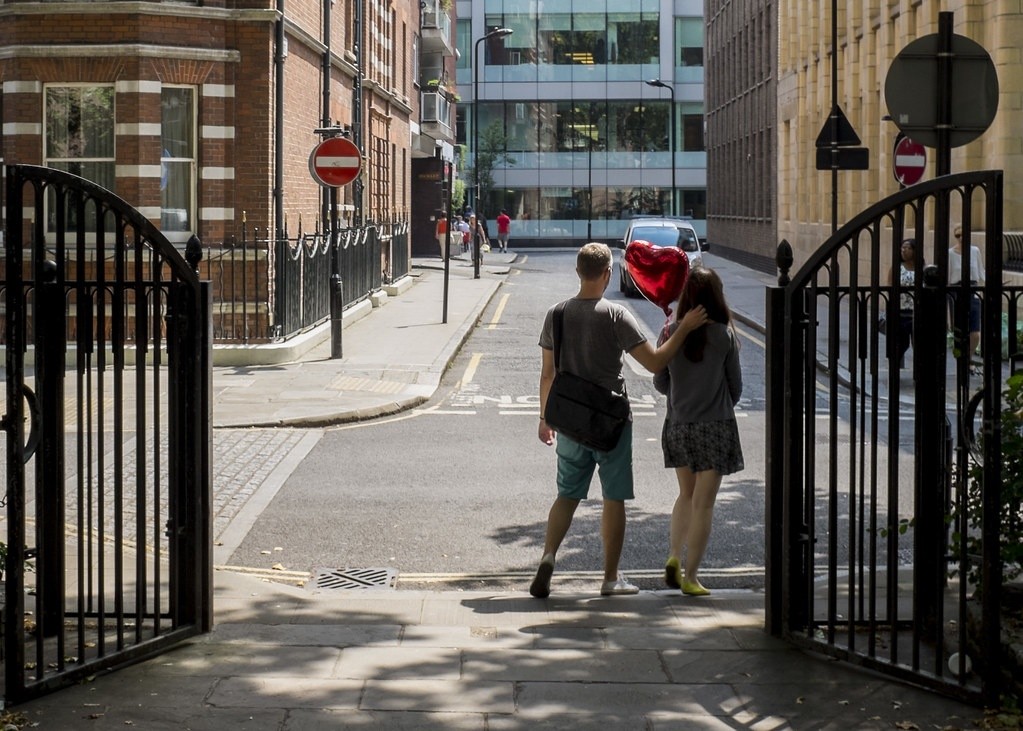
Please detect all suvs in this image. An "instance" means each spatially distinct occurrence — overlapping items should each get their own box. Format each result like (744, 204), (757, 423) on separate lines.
(615, 218), (711, 299)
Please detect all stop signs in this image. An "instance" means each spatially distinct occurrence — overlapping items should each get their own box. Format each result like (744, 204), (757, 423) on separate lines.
(894, 136), (927, 187)
(313, 138), (362, 187)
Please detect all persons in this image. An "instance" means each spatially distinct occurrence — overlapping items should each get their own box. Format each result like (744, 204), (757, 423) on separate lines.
(495, 208), (511, 252)
(887, 238), (926, 370)
(527, 243), (709, 599)
(949, 223), (985, 372)
(652, 268), (744, 594)
(436, 211), (447, 263)
(469, 214), (486, 266)
(450, 204), (493, 252)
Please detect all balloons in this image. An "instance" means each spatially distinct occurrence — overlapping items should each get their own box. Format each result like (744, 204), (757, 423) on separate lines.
(624, 240), (690, 318)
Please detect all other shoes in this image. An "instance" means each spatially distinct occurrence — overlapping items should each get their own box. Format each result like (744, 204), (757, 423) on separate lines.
(499, 247), (507, 253)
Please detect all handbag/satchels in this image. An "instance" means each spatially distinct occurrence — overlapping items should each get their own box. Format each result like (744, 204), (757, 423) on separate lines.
(543, 372), (630, 453)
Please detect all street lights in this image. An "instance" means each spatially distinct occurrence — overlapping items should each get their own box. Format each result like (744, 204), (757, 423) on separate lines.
(473, 28), (514, 280)
(645, 79), (677, 216)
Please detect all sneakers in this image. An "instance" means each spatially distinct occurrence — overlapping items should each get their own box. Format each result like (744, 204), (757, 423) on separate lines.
(665, 556), (682, 589)
(530, 554), (554, 598)
(601, 576), (640, 596)
(681, 576), (711, 595)
